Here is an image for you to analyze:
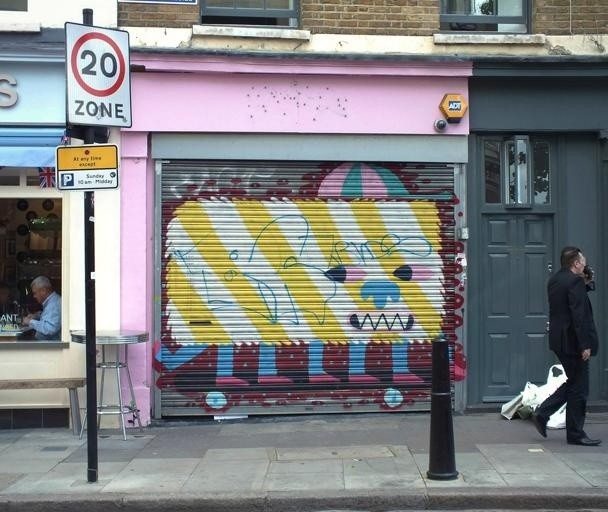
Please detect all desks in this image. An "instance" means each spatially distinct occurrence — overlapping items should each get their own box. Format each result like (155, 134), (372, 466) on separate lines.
(70, 329), (149, 441)
(1, 322), (36, 340)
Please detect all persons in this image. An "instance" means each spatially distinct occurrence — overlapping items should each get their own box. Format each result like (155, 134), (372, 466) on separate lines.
(532, 246), (602, 445)
(23, 275), (61, 340)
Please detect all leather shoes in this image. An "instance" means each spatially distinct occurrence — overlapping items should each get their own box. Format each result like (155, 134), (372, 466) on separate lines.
(532, 406), (550, 438)
(567, 431), (602, 446)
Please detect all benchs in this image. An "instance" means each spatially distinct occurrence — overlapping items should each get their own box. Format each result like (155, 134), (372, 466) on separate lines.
(0, 377), (87, 436)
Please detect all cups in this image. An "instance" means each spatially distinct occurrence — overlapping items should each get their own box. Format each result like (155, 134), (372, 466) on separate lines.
(12, 314), (19, 327)
(583, 264), (593, 280)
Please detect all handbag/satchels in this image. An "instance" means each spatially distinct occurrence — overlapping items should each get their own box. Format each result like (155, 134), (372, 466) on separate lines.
(501, 391), (531, 421)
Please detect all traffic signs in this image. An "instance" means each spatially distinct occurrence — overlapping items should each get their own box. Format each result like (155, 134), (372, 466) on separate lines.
(64, 22), (132, 128)
